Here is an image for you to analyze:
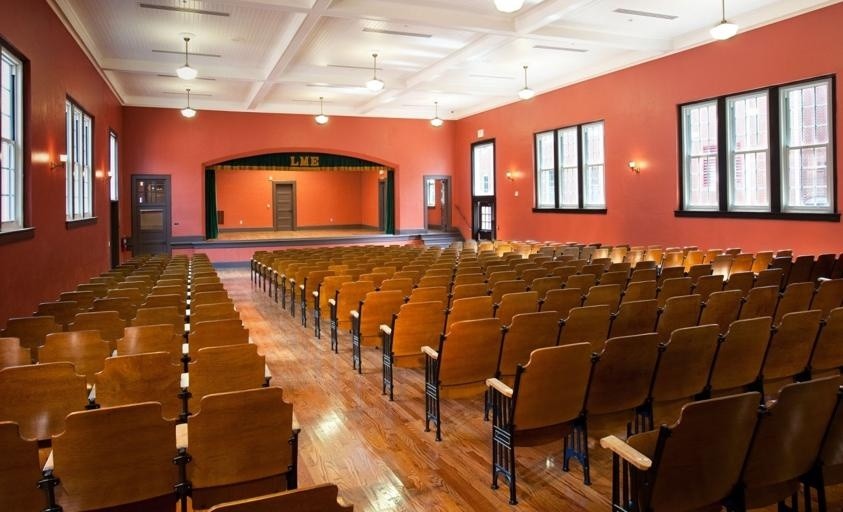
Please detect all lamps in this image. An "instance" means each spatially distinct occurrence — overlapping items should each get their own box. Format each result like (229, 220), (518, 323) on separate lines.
(626, 161), (641, 173)
(314, 96), (329, 124)
(103, 171), (113, 184)
(48, 155), (68, 171)
(517, 65), (535, 99)
(710, 0), (740, 41)
(365, 52), (385, 91)
(431, 102), (444, 128)
(175, 34), (198, 80)
(181, 88), (196, 117)
(505, 171), (513, 182)
(378, 169), (385, 178)
